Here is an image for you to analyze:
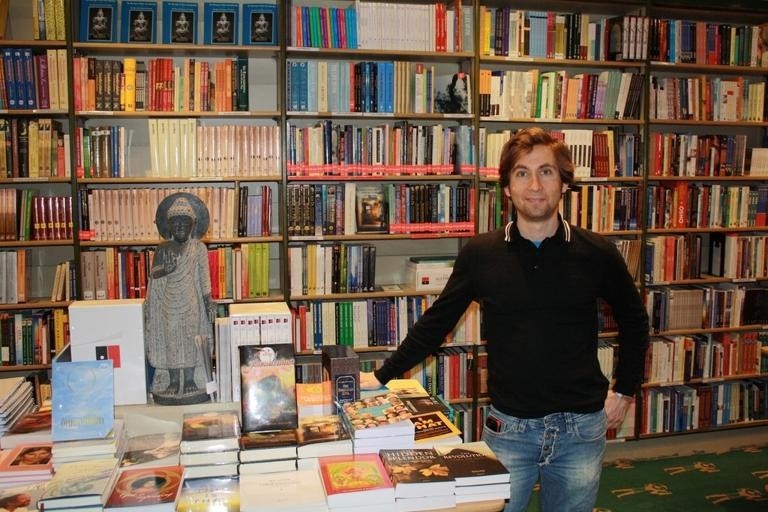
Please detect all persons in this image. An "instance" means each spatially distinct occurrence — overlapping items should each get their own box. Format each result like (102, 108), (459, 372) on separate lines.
(359, 126), (649, 511)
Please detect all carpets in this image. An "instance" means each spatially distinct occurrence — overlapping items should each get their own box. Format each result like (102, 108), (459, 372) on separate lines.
(527, 443), (768, 512)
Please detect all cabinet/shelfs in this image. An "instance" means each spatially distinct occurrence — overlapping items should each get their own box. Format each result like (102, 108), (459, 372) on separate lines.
(1, 1), (766, 443)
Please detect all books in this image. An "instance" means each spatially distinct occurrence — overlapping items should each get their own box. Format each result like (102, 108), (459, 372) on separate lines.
(0, 442), (510, 512)
(476, 2), (767, 442)
(278, 0), (475, 441)
(2, 0), (278, 445)
